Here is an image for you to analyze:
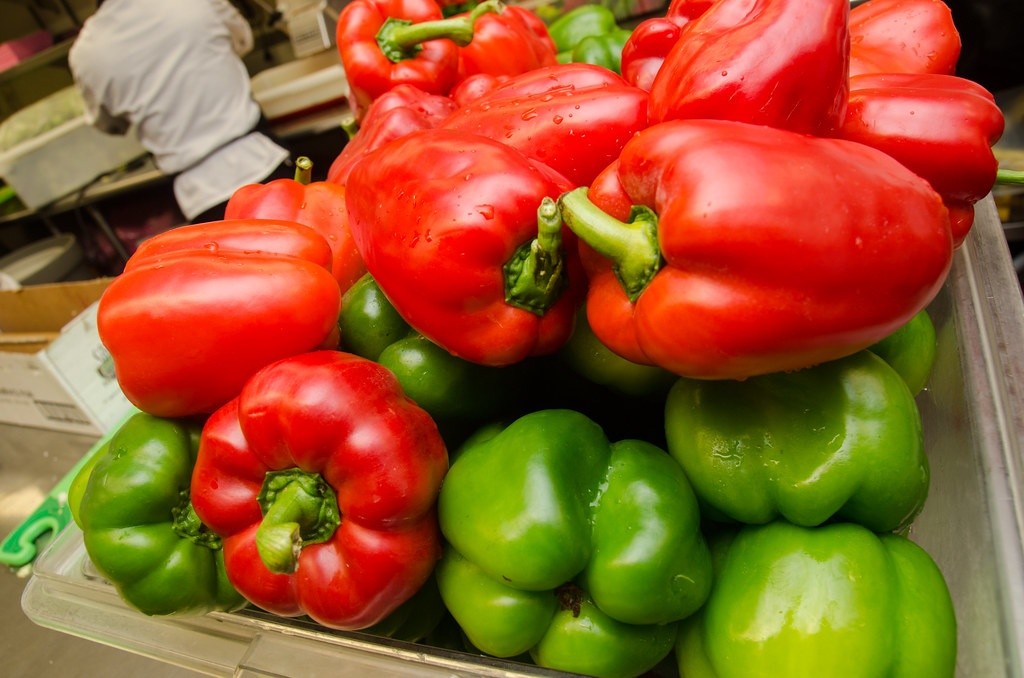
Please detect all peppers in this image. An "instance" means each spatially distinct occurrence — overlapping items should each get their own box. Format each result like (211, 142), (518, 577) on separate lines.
(70, 0), (1004, 678)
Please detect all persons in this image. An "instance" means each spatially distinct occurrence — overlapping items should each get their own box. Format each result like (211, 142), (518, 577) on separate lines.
(69, 0), (291, 225)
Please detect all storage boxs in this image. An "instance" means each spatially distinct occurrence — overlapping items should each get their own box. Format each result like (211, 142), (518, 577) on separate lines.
(0, 278), (124, 438)
(0, 114), (149, 212)
(22, 187), (1024, 677)
